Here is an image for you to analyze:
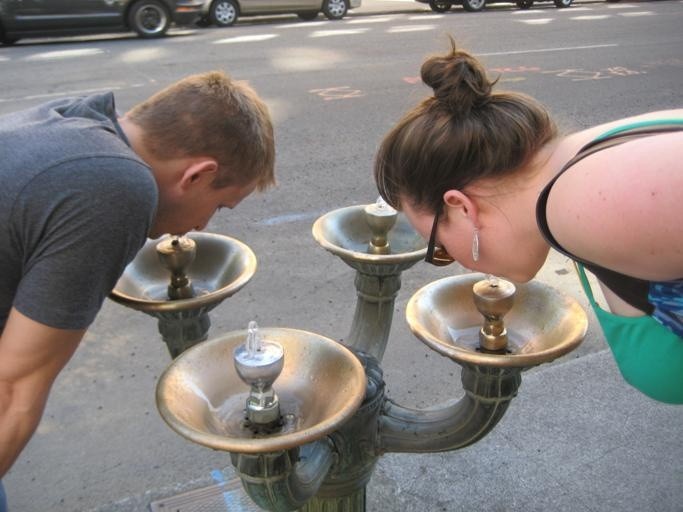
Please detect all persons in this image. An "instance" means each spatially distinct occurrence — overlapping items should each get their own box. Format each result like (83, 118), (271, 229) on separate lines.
(2, 67), (277, 510)
(371, 46), (681, 407)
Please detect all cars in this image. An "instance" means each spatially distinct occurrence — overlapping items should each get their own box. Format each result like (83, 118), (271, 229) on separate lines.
(420, 1), (574, 14)
(173, 0), (362, 29)
(0, 0), (182, 45)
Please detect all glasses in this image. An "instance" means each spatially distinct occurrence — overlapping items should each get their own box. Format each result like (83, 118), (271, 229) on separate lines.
(425, 207), (455, 267)
